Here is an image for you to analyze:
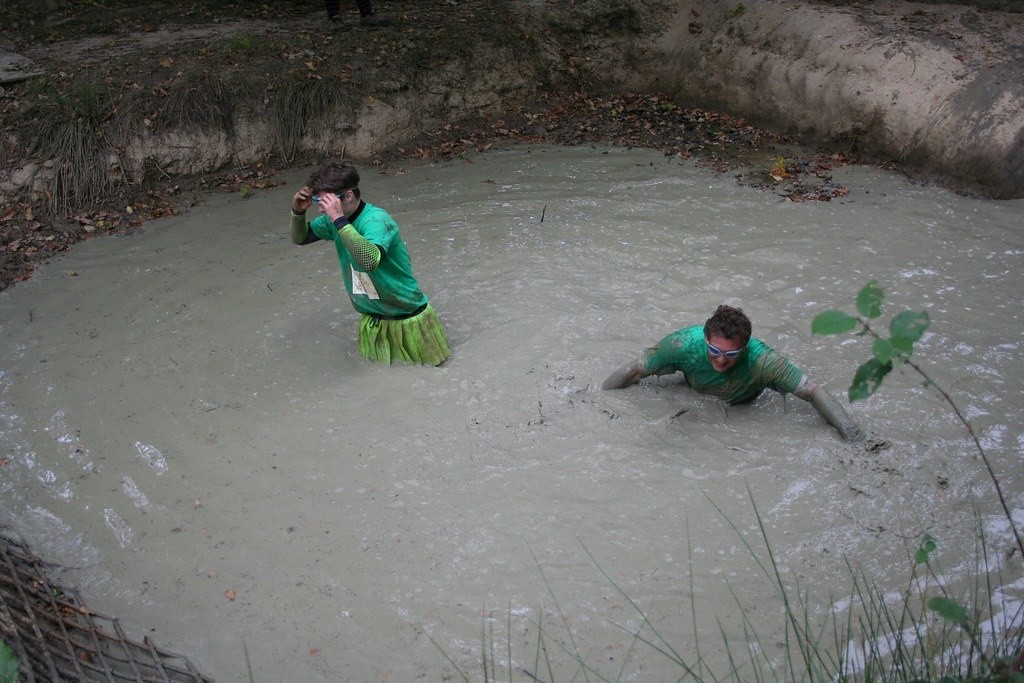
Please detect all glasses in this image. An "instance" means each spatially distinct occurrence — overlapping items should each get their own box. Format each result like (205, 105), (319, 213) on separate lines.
(311, 195), (323, 204)
(707, 339), (744, 359)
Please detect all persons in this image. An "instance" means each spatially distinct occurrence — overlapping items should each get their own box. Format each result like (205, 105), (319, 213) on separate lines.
(290, 160), (452, 366)
(602, 304), (859, 442)
